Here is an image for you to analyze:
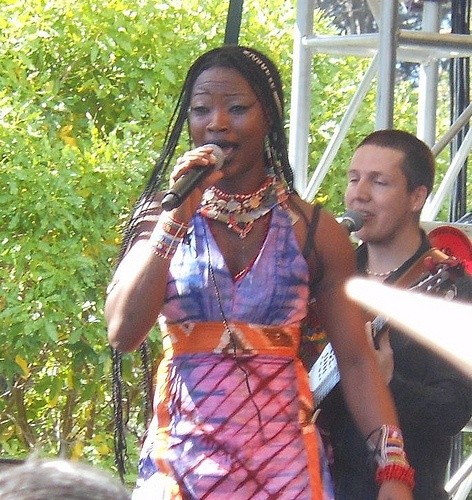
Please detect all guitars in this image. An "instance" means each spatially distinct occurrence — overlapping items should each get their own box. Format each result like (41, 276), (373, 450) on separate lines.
(305, 256), (465, 408)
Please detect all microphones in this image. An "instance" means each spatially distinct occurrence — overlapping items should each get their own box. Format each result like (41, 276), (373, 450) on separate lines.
(335, 212), (364, 236)
(159, 143), (224, 212)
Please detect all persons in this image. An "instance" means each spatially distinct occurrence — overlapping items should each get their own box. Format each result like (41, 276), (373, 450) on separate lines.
(0, 452), (133, 500)
(105, 45), (415, 500)
(302, 129), (472, 500)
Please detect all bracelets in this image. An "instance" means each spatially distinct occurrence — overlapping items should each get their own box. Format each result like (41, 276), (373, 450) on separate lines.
(371, 423), (416, 487)
(148, 211), (190, 261)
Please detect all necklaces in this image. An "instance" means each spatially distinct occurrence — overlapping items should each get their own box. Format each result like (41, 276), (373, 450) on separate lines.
(196, 174), (297, 239)
(359, 264), (402, 278)
